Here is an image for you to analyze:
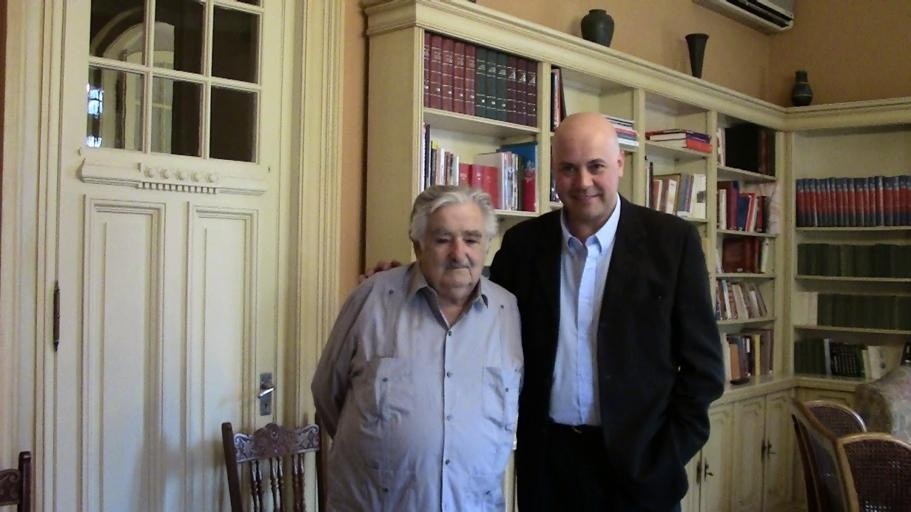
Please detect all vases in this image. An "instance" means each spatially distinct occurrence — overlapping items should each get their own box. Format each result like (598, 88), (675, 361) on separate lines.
(582, 9), (615, 47)
(685, 33), (708, 78)
(791, 70), (813, 107)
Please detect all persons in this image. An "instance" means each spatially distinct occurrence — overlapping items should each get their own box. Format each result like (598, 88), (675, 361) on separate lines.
(310, 182), (527, 512)
(488, 109), (728, 512)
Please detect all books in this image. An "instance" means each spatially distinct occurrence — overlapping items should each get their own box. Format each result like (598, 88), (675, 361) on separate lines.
(717, 122), (775, 177)
(551, 137), (560, 202)
(645, 161), (707, 220)
(424, 30), (538, 128)
(551, 67), (566, 133)
(717, 181), (770, 234)
(715, 235), (776, 387)
(604, 115), (639, 147)
(792, 173), (911, 379)
(645, 128), (713, 153)
(424, 125), (539, 213)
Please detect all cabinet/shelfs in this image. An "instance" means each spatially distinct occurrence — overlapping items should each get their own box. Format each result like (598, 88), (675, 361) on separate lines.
(549, 68), (785, 387)
(364, 27), (549, 282)
(734, 389), (798, 512)
(786, 123), (911, 383)
(680, 405), (733, 512)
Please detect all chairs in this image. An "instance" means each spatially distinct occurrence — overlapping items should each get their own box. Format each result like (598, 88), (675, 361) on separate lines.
(222, 414), (328, 511)
(793, 396), (911, 512)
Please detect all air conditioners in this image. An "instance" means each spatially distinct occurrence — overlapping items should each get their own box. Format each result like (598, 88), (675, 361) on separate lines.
(694, 0), (794, 34)
